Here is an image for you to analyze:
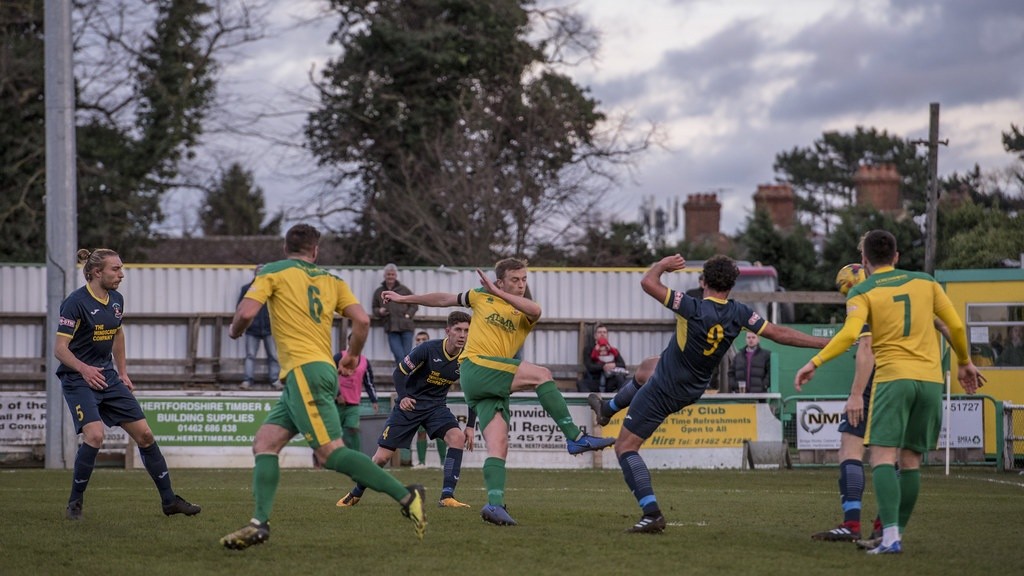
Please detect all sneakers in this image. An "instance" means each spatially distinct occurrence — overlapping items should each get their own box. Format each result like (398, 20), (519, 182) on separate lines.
(336, 492), (360, 508)
(438, 497), (471, 509)
(587, 393), (610, 427)
(66, 507), (80, 521)
(868, 519), (883, 540)
(857, 536), (901, 548)
(623, 513), (665, 534)
(400, 485), (428, 540)
(566, 426), (617, 457)
(162, 495), (201, 516)
(272, 380), (284, 391)
(867, 541), (903, 554)
(219, 519), (270, 551)
(240, 381), (253, 391)
(812, 523), (861, 543)
(480, 502), (519, 526)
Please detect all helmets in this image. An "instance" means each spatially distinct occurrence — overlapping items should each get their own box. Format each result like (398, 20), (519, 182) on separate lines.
(836, 262), (866, 297)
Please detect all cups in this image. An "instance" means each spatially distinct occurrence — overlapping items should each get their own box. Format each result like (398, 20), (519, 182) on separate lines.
(739, 381), (746, 393)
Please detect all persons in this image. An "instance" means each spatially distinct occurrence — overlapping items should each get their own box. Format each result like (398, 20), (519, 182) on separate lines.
(811, 264), (986, 541)
(372, 264), (418, 363)
(381, 259), (615, 525)
(795, 230), (977, 555)
(589, 254), (831, 533)
(583, 325), (628, 392)
(220, 224), (428, 550)
(55, 249), (201, 520)
(334, 333), (378, 450)
(729, 331), (771, 402)
(411, 332), (446, 470)
(710, 343), (736, 390)
(236, 265), (281, 388)
(991, 326), (1024, 366)
(336, 312), (479, 509)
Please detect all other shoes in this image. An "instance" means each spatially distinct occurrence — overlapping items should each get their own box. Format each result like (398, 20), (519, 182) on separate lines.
(411, 462), (427, 470)
(312, 454), (320, 470)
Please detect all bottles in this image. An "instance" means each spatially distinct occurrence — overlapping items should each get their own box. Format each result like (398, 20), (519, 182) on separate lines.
(611, 367), (629, 376)
